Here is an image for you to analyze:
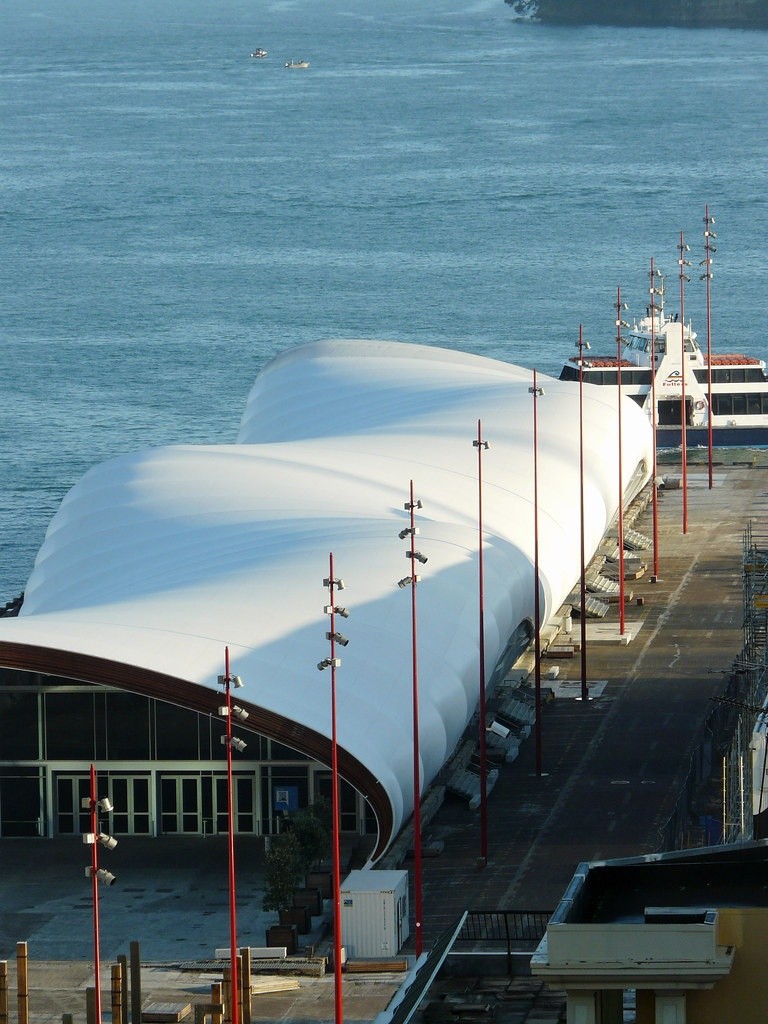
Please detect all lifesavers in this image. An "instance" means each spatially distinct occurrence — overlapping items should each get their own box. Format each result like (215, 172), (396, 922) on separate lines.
(695, 401), (705, 410)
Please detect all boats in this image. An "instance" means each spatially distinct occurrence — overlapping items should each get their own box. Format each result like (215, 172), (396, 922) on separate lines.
(284, 59), (311, 68)
(558, 272), (768, 450)
(250, 48), (268, 58)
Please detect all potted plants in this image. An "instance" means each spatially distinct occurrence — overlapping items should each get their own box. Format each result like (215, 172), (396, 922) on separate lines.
(262, 793), (332, 954)
